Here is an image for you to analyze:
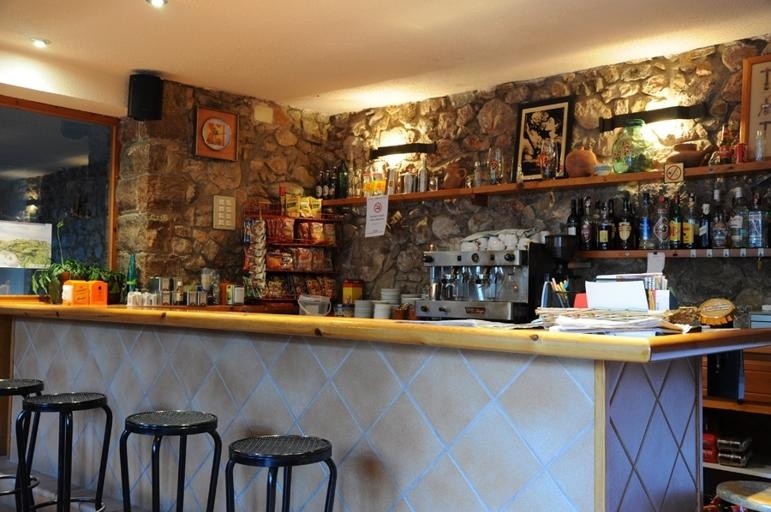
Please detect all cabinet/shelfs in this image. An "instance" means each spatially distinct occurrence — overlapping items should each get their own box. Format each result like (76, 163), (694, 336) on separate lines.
(703, 397), (771, 479)
(242, 206), (343, 301)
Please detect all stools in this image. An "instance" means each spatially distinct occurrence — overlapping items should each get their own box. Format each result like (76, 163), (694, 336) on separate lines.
(15, 392), (112, 512)
(0, 378), (43, 496)
(119, 410), (222, 512)
(717, 480), (771, 512)
(225, 434), (337, 512)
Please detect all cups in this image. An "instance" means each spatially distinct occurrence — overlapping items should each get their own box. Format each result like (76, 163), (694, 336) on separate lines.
(474, 145), (506, 186)
(540, 140), (560, 178)
(461, 229), (551, 252)
(354, 288), (415, 319)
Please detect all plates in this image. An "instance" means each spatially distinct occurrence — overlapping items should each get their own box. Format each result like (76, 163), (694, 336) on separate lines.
(202, 117), (233, 151)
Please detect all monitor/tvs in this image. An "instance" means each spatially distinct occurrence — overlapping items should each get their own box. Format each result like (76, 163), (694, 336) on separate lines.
(0, 218), (55, 272)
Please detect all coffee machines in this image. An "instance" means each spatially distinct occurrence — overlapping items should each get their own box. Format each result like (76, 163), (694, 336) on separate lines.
(416, 243), (553, 322)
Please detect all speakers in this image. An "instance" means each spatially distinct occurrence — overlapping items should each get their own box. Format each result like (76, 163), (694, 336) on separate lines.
(128, 74), (164, 122)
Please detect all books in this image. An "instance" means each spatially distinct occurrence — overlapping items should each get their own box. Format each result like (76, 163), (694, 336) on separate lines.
(533, 304), (683, 336)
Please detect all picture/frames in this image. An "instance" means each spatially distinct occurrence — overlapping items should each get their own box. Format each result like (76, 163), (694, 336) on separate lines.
(510, 95), (576, 182)
(739, 53), (771, 159)
(193, 104), (239, 162)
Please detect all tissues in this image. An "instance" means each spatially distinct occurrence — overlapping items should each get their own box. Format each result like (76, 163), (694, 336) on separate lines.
(61, 279), (110, 306)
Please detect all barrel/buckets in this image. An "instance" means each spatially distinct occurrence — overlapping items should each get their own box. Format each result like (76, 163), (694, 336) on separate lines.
(298, 294), (332, 317)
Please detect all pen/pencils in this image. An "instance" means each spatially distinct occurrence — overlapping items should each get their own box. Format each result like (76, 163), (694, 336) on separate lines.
(549, 277), (570, 308)
(645, 275), (668, 291)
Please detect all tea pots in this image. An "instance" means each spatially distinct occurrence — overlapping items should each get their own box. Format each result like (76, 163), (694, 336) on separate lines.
(440, 163), (467, 190)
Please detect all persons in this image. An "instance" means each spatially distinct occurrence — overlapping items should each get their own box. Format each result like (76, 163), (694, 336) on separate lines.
(522, 112), (558, 162)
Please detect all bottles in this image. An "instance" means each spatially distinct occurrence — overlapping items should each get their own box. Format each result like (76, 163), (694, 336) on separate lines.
(753, 130), (768, 161)
(313, 154), (430, 197)
(719, 122), (732, 162)
(568, 189), (768, 248)
(127, 253), (137, 293)
(176, 281), (184, 305)
